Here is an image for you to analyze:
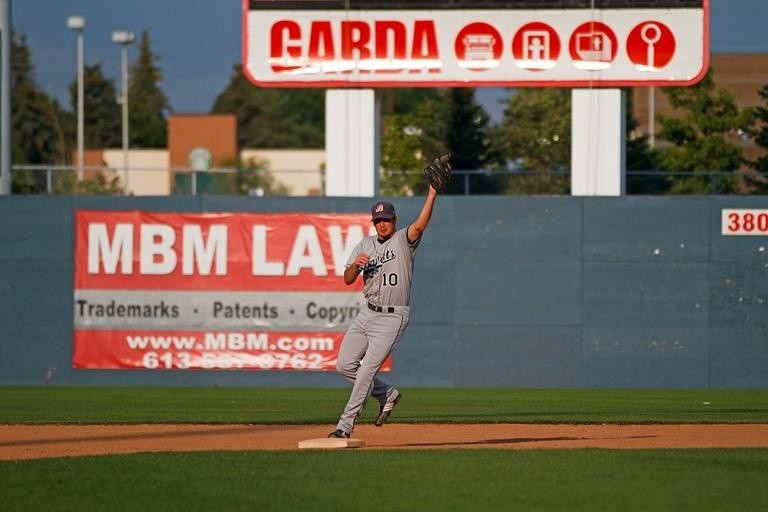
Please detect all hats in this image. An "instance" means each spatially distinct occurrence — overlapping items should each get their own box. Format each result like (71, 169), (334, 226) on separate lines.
(371, 202), (395, 222)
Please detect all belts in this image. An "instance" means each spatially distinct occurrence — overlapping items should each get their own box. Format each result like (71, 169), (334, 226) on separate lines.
(366, 302), (394, 313)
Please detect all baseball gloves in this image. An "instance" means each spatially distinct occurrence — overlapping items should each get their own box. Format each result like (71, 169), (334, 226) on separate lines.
(425, 152), (454, 194)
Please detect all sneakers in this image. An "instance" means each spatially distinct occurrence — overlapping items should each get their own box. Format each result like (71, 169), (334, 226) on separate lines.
(328, 429), (350, 438)
(375, 387), (400, 426)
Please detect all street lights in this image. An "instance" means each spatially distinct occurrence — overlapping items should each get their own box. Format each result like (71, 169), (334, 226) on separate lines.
(67, 16), (86, 182)
(111, 31), (136, 199)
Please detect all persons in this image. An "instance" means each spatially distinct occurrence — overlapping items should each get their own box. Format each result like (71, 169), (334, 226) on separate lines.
(323, 149), (454, 440)
